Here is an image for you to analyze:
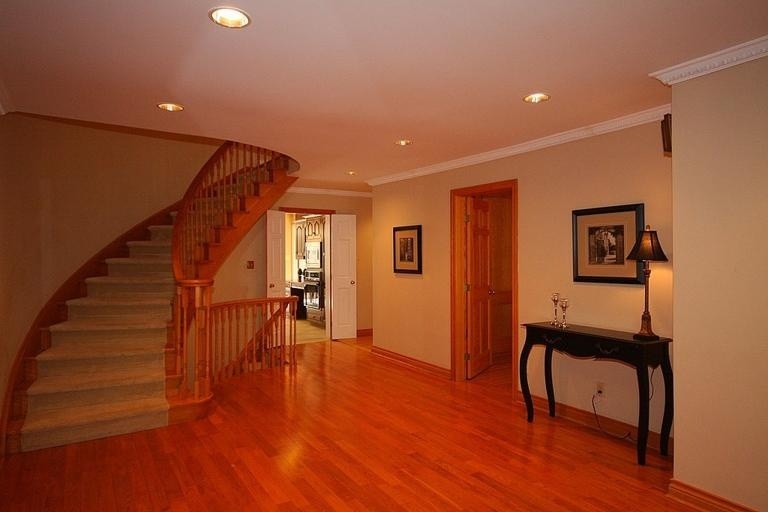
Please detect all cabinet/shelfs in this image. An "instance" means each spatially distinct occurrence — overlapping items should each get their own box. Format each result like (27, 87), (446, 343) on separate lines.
(294, 220), (306, 259)
(306, 217), (323, 243)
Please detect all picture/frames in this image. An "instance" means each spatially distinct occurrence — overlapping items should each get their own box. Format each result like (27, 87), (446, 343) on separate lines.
(570, 201), (649, 288)
(391, 224), (423, 275)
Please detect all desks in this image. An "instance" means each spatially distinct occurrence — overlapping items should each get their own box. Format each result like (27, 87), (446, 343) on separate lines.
(520, 319), (673, 467)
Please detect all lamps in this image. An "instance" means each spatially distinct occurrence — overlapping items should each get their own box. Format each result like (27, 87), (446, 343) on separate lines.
(625, 224), (669, 342)
